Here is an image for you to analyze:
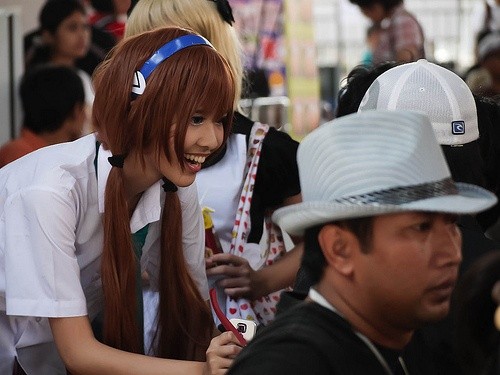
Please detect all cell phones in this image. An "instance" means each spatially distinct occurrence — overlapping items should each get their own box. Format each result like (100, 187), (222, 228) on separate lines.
(229, 319), (257, 344)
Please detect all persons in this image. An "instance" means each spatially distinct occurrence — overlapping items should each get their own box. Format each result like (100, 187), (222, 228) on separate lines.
(0, 0), (128, 171)
(0, 26), (247, 375)
(226, 109), (499, 375)
(124, 0), (307, 332)
(336, 0), (500, 277)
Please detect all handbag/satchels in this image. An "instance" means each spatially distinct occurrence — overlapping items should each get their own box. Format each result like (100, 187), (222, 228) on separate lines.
(224, 118), (301, 333)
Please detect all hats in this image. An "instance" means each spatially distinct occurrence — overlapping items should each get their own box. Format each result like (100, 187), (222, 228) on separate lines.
(354, 58), (479, 146)
(272, 110), (497, 236)
